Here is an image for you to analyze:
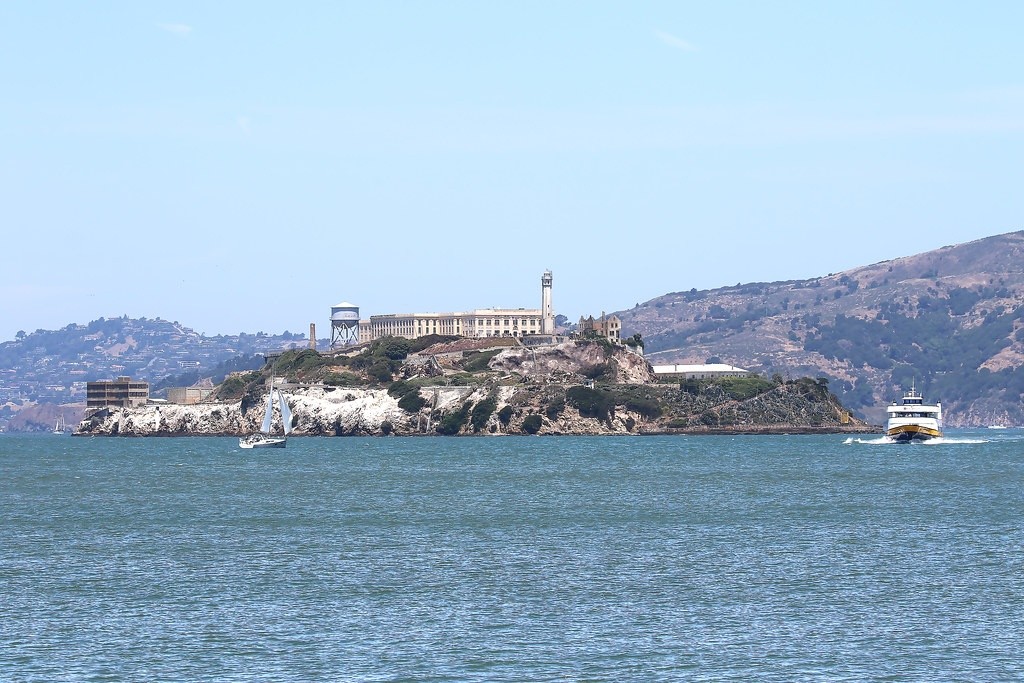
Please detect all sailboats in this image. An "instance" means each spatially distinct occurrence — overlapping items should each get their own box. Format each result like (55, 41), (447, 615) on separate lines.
(238, 376), (294, 449)
(53, 411), (65, 435)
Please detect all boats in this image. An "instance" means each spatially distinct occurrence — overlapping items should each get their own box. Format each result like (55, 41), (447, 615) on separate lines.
(885, 374), (944, 444)
(988, 425), (1007, 429)
